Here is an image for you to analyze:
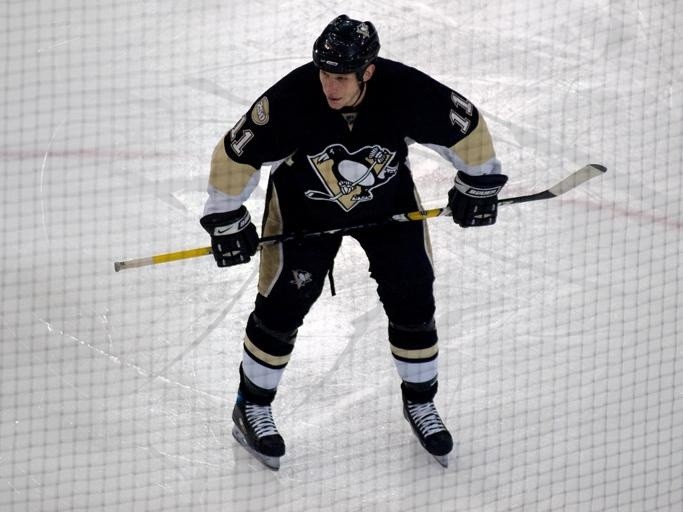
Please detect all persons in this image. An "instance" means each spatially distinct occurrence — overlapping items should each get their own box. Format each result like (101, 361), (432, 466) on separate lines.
(194, 14), (509, 454)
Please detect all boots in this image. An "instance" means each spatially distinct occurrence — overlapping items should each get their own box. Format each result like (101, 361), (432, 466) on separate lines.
(400, 372), (453, 456)
(231, 360), (286, 458)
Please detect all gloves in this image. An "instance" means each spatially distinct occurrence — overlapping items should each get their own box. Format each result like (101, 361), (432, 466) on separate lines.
(444, 170), (509, 228)
(199, 204), (259, 268)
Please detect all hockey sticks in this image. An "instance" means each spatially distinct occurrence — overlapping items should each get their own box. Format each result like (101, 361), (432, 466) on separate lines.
(114, 163), (607, 273)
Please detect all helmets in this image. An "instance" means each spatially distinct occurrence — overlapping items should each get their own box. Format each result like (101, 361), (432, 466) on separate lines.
(312, 14), (381, 83)
